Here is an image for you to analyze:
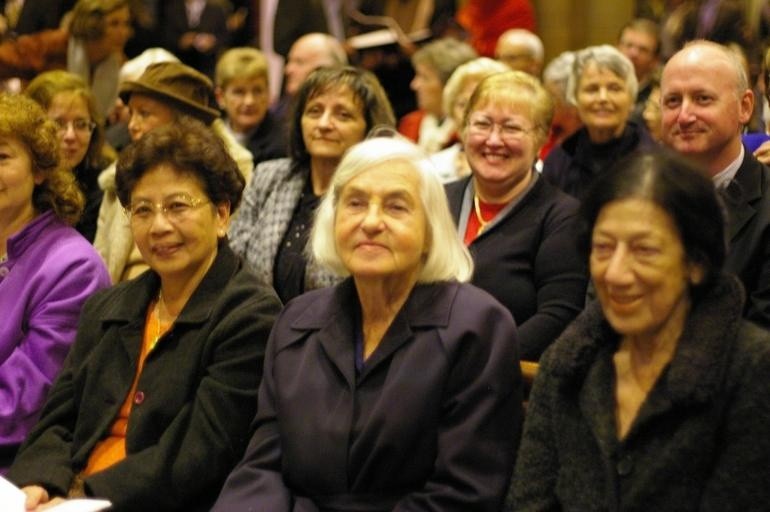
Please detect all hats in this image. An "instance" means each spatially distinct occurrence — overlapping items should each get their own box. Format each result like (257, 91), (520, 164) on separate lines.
(119, 62), (220, 125)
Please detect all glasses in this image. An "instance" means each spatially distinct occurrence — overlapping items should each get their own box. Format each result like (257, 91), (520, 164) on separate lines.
(464, 116), (539, 139)
(123, 194), (210, 220)
(53, 119), (96, 134)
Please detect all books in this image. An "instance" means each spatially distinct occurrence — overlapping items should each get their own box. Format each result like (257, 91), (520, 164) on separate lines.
(351, 28), (432, 52)
(0, 476), (113, 512)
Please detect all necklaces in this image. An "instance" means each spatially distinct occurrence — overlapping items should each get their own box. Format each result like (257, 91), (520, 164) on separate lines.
(473, 189), (489, 237)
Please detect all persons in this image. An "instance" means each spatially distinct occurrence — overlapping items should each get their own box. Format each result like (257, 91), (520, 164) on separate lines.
(452, 0), (535, 56)
(429, 55), (545, 184)
(213, 45), (289, 165)
(90, 59), (223, 284)
(38, 0), (135, 122)
(540, 43), (663, 204)
(269, 31), (350, 122)
(502, 141), (770, 511)
(1, 0), (73, 92)
(206, 134), (522, 509)
(660, 0), (763, 90)
(165, 1), (249, 81)
(616, 17), (661, 142)
(752, 139), (770, 165)
(273, 0), (360, 100)
(536, 49), (583, 162)
(494, 27), (545, 79)
(226, 64), (398, 300)
(655, 36), (770, 331)
(0, 91), (114, 476)
(443, 66), (594, 360)
(398, 35), (482, 154)
(101, 46), (181, 151)
(361, 0), (444, 81)
(4, 114), (286, 511)
(22, 67), (115, 245)
